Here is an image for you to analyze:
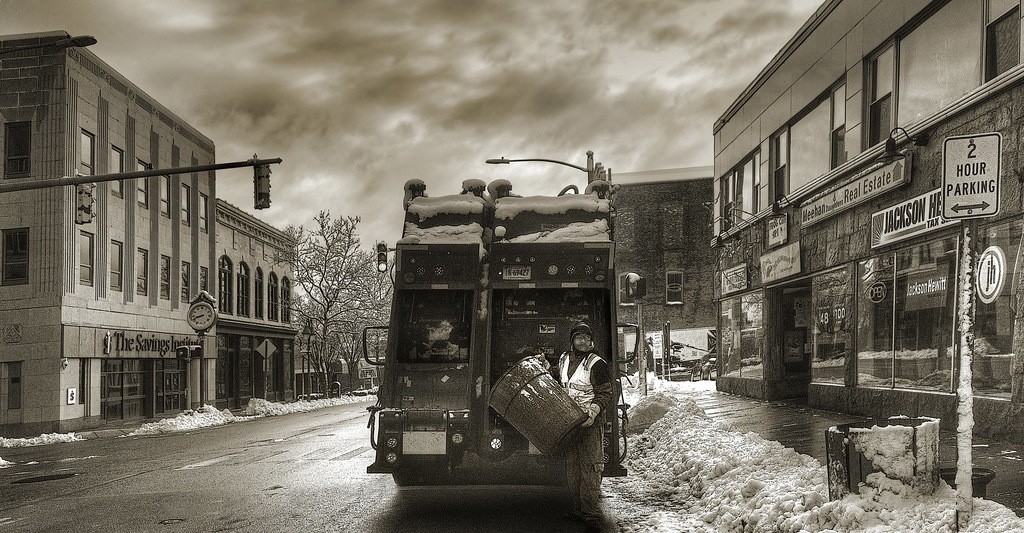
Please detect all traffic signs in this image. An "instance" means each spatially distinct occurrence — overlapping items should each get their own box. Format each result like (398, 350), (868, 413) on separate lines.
(940, 132), (1004, 221)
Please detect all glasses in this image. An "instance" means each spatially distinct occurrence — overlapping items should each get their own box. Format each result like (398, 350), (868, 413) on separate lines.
(575, 335), (591, 341)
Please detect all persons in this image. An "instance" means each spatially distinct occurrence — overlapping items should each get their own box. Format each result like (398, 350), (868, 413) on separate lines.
(535, 323), (612, 533)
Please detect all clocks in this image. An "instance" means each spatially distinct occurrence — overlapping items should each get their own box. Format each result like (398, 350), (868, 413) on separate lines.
(187, 301), (218, 332)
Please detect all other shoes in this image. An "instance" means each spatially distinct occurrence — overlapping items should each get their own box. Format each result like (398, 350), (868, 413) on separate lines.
(562, 512), (583, 521)
(585, 515), (601, 530)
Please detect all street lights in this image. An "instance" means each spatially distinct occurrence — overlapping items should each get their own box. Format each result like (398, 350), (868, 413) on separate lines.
(302, 318), (316, 401)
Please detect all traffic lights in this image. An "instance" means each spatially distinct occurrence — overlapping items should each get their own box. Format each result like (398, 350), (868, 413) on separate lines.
(376, 242), (388, 273)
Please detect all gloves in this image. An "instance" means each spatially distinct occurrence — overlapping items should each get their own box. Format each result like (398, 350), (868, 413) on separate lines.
(535, 353), (551, 371)
(581, 402), (600, 427)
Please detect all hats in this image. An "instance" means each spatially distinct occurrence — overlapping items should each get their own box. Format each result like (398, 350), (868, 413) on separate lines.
(570, 320), (593, 344)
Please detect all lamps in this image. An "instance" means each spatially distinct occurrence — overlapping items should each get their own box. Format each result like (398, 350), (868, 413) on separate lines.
(767, 194), (801, 221)
(875, 128), (929, 165)
(712, 230), (741, 250)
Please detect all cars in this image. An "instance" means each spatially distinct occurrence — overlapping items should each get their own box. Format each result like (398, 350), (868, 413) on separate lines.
(700, 358), (729, 381)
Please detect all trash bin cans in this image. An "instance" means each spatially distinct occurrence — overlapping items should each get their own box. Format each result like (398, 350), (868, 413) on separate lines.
(823, 415), (941, 503)
(330, 381), (341, 398)
(486, 354), (590, 460)
(942, 465), (996, 500)
